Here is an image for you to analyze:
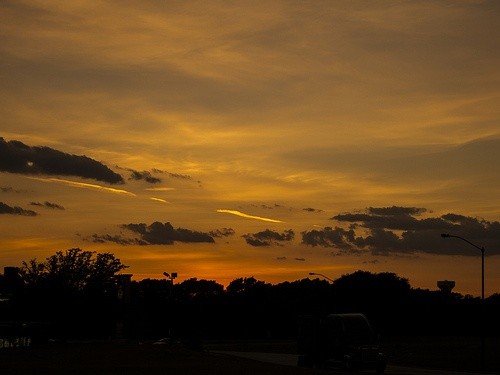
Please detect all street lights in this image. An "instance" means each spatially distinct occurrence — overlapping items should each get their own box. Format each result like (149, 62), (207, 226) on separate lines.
(309, 273), (335, 283)
(441, 233), (484, 304)
(165, 271), (177, 342)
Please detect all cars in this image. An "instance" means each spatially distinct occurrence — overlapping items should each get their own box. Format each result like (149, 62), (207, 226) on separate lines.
(298, 310), (385, 373)
